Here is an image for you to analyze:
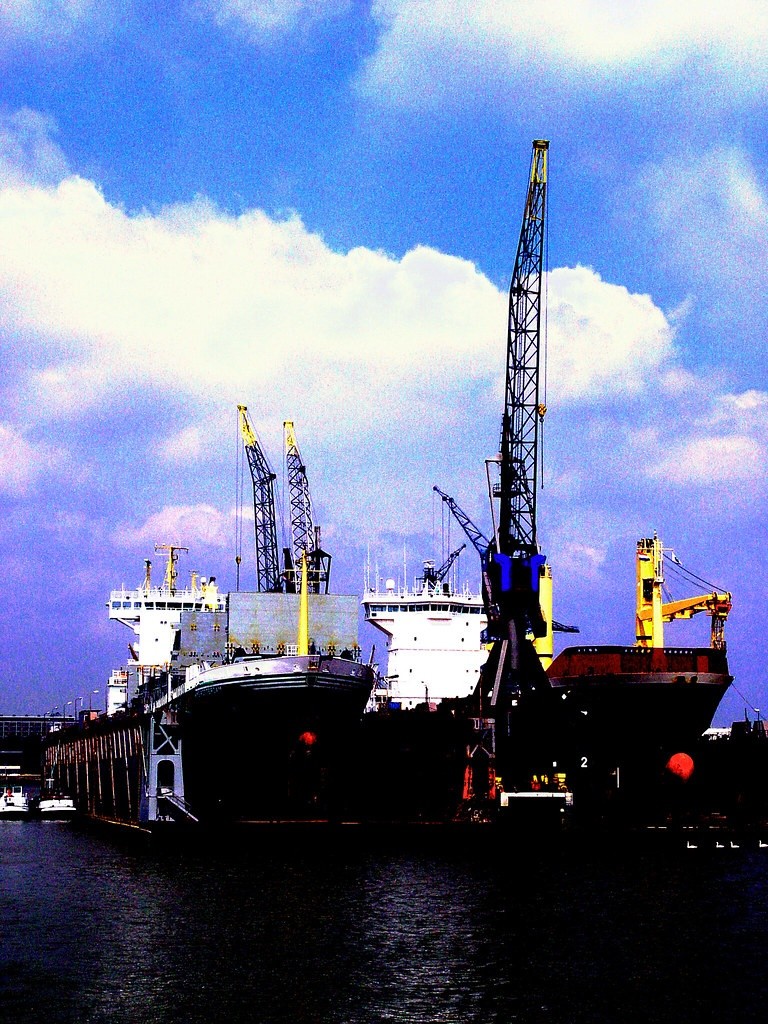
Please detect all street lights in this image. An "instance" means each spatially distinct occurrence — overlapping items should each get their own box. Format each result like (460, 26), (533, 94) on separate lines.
(75, 697), (83, 721)
(51, 706), (58, 731)
(43, 712), (51, 728)
(63, 702), (73, 728)
(90, 689), (100, 710)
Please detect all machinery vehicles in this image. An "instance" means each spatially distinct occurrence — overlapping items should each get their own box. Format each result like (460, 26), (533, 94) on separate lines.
(231, 404), (333, 594)
(429, 137), (734, 696)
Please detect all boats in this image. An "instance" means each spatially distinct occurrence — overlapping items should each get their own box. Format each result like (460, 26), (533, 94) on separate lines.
(0, 786), (32, 814)
(41, 525), (766, 836)
(32, 778), (77, 818)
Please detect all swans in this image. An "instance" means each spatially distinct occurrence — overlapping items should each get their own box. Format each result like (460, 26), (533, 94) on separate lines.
(686, 840), (768, 849)
